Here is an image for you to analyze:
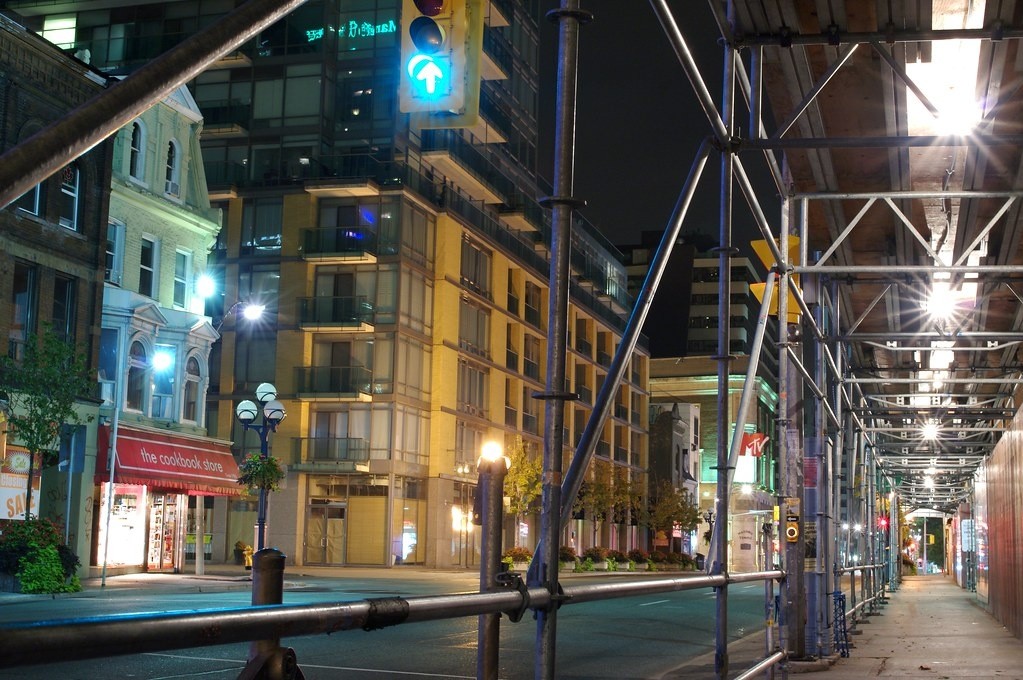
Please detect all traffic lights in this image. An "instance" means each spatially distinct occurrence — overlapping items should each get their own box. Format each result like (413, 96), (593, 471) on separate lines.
(399, 0), (465, 113)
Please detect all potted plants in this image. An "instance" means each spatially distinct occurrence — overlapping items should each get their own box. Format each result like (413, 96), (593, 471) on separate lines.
(499, 545), (697, 576)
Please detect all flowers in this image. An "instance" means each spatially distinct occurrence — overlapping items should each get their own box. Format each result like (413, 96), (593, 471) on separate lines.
(237, 451), (287, 493)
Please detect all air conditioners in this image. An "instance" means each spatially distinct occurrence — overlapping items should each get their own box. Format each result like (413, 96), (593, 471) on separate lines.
(164, 181), (180, 199)
(104, 269), (121, 286)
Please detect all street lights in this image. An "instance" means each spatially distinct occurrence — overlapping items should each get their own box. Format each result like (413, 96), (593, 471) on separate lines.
(703, 508), (716, 542)
(236, 383), (287, 551)
